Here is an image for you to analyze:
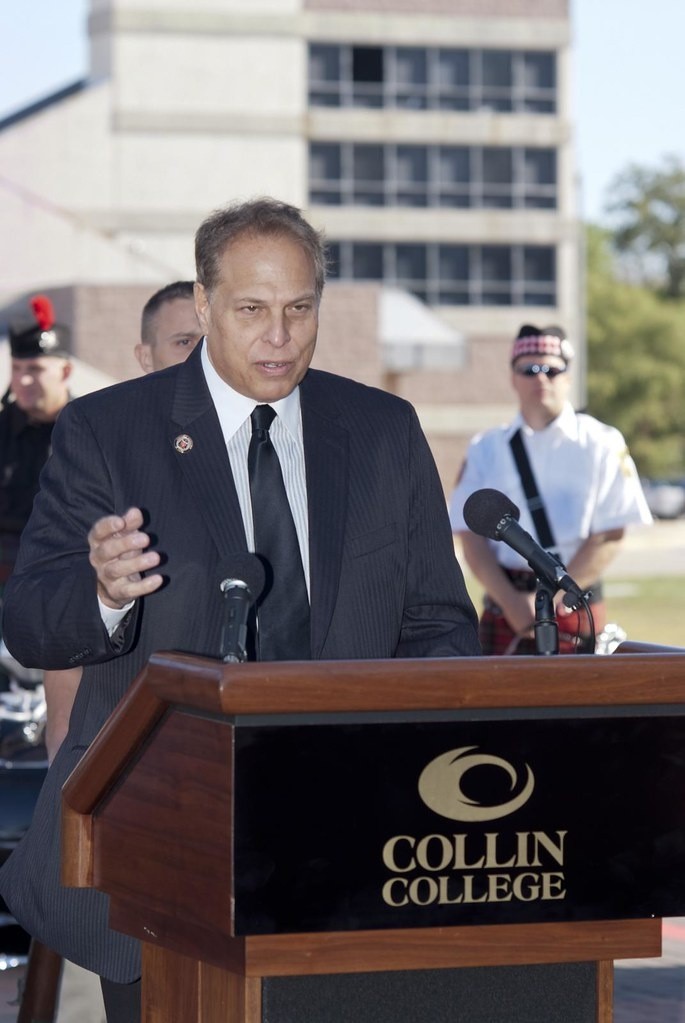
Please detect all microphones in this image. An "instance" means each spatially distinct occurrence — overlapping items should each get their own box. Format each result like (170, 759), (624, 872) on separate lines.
(464, 488), (585, 601)
(216, 552), (266, 666)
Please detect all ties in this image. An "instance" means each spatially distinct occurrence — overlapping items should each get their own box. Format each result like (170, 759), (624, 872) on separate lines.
(247, 404), (309, 661)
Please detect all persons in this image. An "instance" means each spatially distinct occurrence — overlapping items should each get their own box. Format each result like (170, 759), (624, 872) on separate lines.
(40, 280), (205, 763)
(447, 325), (653, 656)
(0, 321), (76, 595)
(0, 198), (478, 1023)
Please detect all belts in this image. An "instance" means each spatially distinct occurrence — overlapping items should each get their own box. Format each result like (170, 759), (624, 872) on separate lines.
(502, 567), (537, 589)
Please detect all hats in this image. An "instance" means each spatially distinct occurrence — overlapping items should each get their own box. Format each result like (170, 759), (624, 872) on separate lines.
(512, 325), (573, 364)
(8, 295), (69, 358)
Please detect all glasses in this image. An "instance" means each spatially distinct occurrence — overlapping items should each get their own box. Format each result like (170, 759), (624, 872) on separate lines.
(515, 365), (565, 378)
